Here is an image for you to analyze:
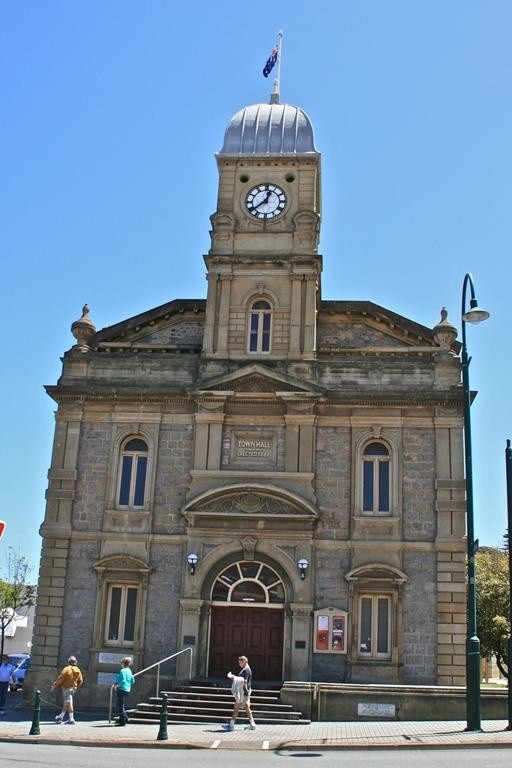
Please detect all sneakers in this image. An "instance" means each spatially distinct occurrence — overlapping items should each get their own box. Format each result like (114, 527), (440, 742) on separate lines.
(221, 725), (234, 731)
(54, 715), (62, 724)
(64, 720), (75, 724)
(244, 724), (255, 730)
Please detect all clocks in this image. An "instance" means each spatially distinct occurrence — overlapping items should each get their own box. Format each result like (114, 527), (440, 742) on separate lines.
(244, 182), (286, 219)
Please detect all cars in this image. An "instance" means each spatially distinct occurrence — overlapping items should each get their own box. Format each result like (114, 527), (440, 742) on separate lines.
(6, 654), (30, 668)
(9, 657), (31, 692)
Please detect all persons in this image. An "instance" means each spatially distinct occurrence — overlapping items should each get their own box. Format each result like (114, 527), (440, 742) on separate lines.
(51, 655), (83, 724)
(113, 657), (135, 726)
(0, 654), (16, 715)
(221, 656), (256, 731)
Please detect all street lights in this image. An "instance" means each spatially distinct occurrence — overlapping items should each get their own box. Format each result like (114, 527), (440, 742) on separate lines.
(459, 271), (490, 730)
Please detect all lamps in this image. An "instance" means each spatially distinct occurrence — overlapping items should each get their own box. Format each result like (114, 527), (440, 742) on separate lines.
(187, 554), (199, 575)
(297, 559), (310, 580)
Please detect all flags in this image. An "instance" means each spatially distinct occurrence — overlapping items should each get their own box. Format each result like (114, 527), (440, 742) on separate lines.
(263, 43), (279, 77)
